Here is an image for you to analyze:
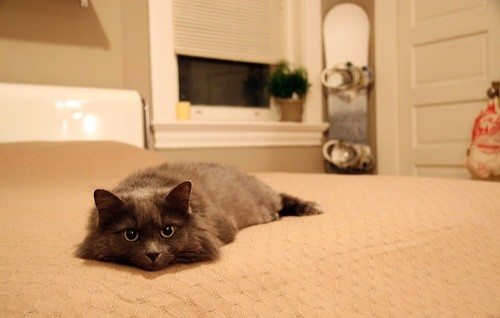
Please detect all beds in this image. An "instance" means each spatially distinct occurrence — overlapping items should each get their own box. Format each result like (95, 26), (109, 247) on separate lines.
(0, 82), (500, 318)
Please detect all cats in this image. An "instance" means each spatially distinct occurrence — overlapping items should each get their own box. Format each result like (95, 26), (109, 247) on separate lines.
(72, 160), (323, 271)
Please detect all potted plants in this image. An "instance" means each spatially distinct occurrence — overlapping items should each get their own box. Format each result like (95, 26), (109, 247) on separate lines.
(265, 59), (311, 122)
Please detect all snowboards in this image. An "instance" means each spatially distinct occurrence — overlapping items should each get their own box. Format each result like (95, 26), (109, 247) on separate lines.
(321, 3), (374, 174)
